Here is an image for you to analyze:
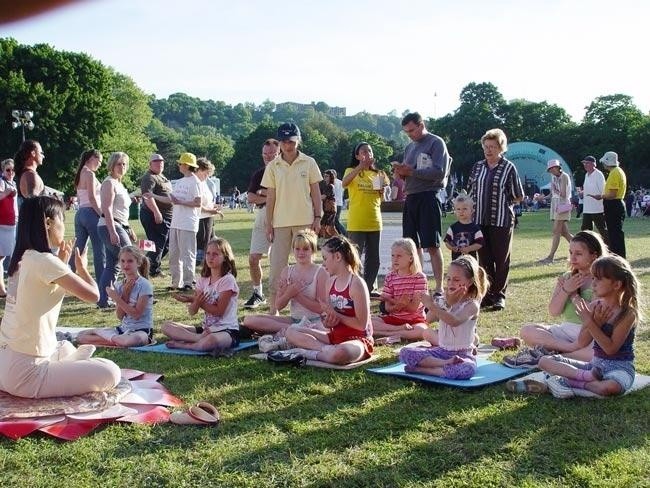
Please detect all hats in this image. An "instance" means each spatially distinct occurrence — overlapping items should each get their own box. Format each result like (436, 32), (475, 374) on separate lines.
(547, 160), (561, 173)
(175, 152), (199, 168)
(600, 151), (620, 166)
(151, 154), (164, 161)
(584, 155), (596, 162)
(277, 123), (300, 142)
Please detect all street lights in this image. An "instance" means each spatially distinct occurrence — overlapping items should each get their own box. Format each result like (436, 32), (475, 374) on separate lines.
(11, 109), (35, 143)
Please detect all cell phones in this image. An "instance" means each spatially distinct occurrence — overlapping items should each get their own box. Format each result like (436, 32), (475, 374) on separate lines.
(390, 161), (400, 166)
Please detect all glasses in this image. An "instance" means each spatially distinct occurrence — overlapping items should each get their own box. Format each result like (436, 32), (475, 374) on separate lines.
(40, 151), (44, 154)
(261, 153), (276, 156)
(583, 162), (589, 166)
(323, 174), (330, 177)
(6, 169), (15, 172)
(177, 162), (188, 166)
(482, 144), (499, 150)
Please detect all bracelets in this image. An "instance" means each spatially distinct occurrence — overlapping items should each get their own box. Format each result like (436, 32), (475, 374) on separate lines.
(437, 300), (453, 312)
(554, 189), (559, 193)
(601, 195), (602, 199)
(314, 216), (322, 219)
(562, 286), (572, 296)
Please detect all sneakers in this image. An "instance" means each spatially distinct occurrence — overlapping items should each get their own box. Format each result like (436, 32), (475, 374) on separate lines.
(370, 293), (381, 299)
(492, 337), (521, 350)
(183, 285), (192, 291)
(540, 258), (553, 263)
(244, 290), (265, 308)
(168, 287), (184, 291)
(545, 375), (575, 399)
(97, 302), (116, 311)
(273, 328), (287, 349)
(375, 335), (401, 345)
(534, 345), (556, 357)
(258, 334), (278, 352)
(151, 272), (168, 280)
(268, 351), (291, 362)
(506, 371), (547, 392)
(493, 297), (505, 311)
(433, 293), (444, 298)
(290, 353), (303, 361)
(503, 349), (542, 369)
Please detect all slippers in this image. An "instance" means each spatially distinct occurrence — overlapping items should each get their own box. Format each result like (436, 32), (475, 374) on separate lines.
(195, 402), (220, 421)
(169, 406), (218, 424)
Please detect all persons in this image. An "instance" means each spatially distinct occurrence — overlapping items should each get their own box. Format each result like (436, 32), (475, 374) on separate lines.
(0, 197), (121, 399)
(321, 171), (340, 238)
(206, 163), (222, 238)
(571, 186), (583, 218)
(534, 159), (574, 264)
(77, 246), (153, 346)
(342, 142), (385, 299)
(69, 149), (106, 285)
(438, 184), (467, 217)
(512, 193), (549, 228)
(193, 158), (225, 267)
(371, 237), (428, 340)
(390, 169), (406, 200)
(141, 152), (201, 290)
(96, 152), (158, 307)
(467, 129), (525, 311)
(393, 112), (449, 297)
(443, 195), (484, 261)
(244, 138), (281, 309)
(162, 237), (240, 352)
(243, 228), (329, 334)
(519, 230), (609, 362)
(400, 254), (494, 380)
(382, 171), (392, 200)
(284, 236), (374, 365)
(328, 169), (347, 235)
(591, 151), (626, 258)
(216, 186), (257, 213)
(578, 156), (609, 245)
(0, 158), (19, 298)
(14, 139), (61, 256)
(48, 192), (78, 211)
(260, 123), (324, 316)
(139, 154), (173, 277)
(624, 186), (650, 217)
(538, 252), (642, 396)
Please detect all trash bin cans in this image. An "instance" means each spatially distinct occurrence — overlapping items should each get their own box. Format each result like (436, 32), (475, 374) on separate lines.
(129, 202), (138, 219)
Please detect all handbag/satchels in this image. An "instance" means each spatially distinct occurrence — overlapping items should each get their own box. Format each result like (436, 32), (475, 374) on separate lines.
(123, 226), (132, 235)
(57, 331), (72, 341)
(324, 197), (337, 213)
(239, 325), (264, 341)
(43, 186), (65, 202)
(0, 191), (15, 225)
(391, 186), (398, 200)
(513, 204), (522, 216)
(556, 193), (572, 215)
(416, 152), (453, 177)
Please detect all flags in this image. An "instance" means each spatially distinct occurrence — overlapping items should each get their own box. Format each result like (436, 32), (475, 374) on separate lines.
(139, 239), (156, 252)
